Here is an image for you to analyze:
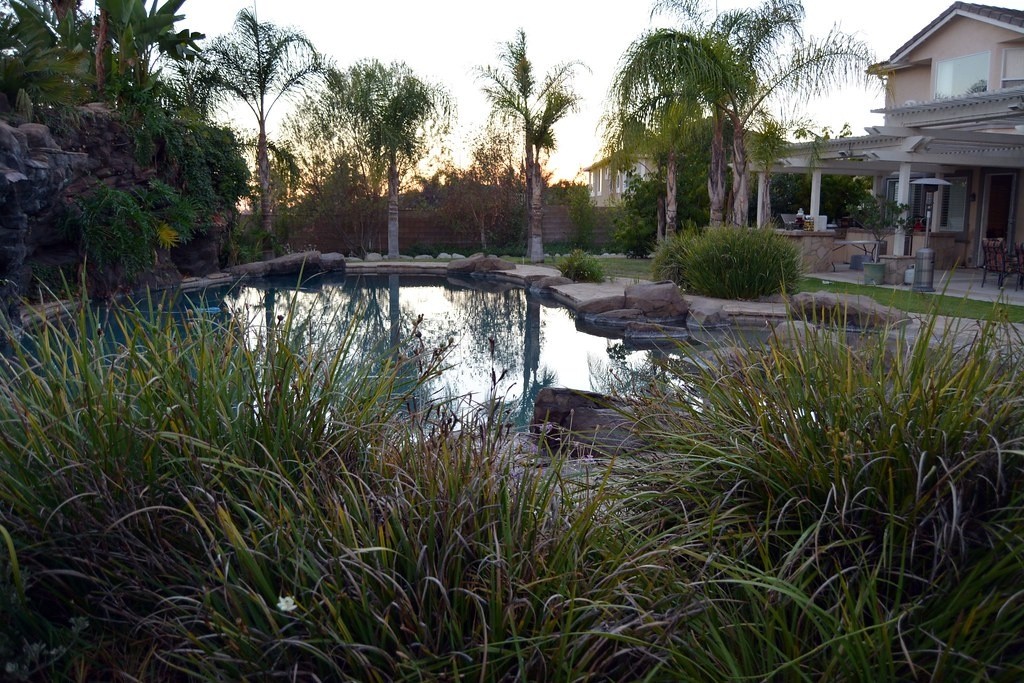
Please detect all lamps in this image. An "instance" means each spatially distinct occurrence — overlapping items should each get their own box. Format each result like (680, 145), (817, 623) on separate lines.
(838, 150), (852, 156)
(1008, 96), (1024, 111)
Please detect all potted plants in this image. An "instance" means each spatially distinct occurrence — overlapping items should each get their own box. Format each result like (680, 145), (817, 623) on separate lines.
(845, 193), (918, 285)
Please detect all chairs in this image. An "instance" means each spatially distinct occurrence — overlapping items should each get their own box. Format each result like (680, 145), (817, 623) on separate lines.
(981, 237), (1019, 290)
(1014, 241), (1024, 292)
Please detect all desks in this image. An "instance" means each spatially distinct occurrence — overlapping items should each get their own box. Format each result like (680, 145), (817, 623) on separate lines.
(832, 239), (885, 272)
(997, 250), (1019, 285)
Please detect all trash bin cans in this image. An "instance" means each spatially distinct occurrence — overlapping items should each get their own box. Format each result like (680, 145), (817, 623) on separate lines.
(912, 248), (936, 292)
(879, 239), (887, 255)
